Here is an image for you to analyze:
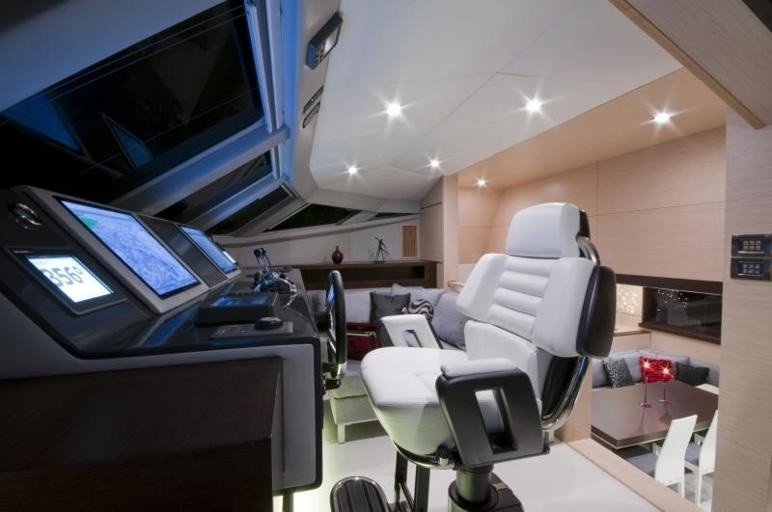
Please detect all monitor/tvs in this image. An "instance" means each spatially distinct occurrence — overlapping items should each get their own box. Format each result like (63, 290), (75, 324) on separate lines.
(20, 185), (242, 314)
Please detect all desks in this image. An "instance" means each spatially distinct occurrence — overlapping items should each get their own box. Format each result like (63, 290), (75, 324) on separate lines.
(590, 380), (719, 451)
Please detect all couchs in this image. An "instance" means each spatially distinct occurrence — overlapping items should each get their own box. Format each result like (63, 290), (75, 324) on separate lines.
(591, 348), (720, 398)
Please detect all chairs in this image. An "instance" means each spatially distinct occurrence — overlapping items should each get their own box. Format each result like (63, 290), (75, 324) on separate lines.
(627, 410), (718, 507)
(359, 201), (617, 512)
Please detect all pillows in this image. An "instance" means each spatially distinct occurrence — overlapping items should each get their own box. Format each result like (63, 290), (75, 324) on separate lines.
(591, 352), (709, 386)
(369, 283), (470, 350)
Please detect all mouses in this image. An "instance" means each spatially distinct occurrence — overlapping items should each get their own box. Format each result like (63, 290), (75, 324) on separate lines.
(255, 316), (283, 330)
